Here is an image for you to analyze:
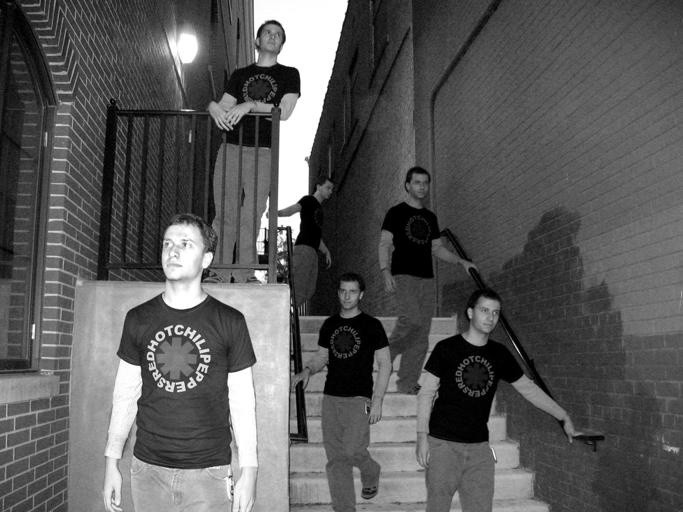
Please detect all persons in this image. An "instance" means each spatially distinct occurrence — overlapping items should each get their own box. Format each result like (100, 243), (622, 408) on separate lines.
(103, 214), (258, 512)
(266, 176), (334, 308)
(291, 273), (392, 512)
(416, 289), (584, 512)
(202, 20), (300, 283)
(378, 166), (477, 395)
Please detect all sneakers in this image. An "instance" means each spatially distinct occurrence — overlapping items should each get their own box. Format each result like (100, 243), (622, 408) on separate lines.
(361, 487), (379, 500)
(202, 272), (262, 283)
